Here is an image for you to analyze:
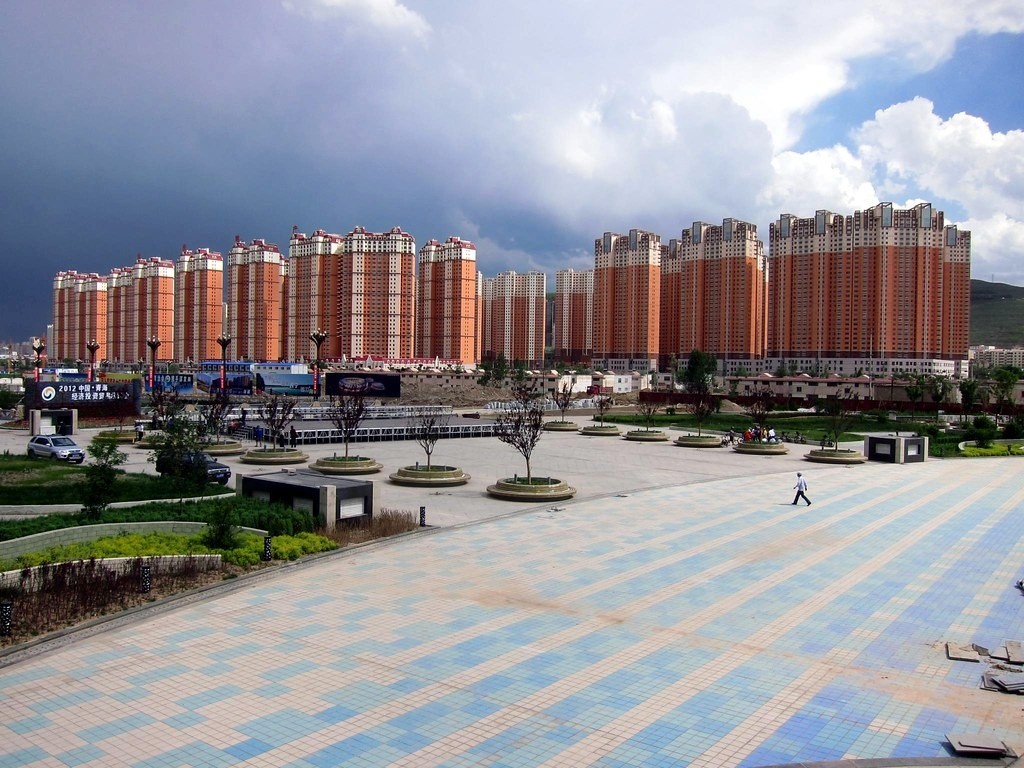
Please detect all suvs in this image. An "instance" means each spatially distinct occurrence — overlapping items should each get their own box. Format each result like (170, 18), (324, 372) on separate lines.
(155, 445), (232, 487)
(26, 434), (85, 464)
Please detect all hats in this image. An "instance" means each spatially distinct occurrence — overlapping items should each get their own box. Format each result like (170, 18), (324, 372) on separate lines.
(748, 427), (752, 429)
(797, 472), (802, 475)
(731, 426), (734, 428)
(764, 428), (765, 429)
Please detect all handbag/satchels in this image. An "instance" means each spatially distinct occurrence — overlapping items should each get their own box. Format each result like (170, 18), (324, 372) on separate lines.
(295, 432), (299, 438)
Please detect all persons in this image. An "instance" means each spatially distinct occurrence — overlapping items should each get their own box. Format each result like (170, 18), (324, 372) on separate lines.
(278, 425), (299, 448)
(791, 473), (811, 506)
(255, 425), (262, 447)
(137, 423), (143, 441)
(166, 415), (173, 433)
(219, 408), (248, 435)
(727, 425), (776, 443)
(152, 413), (158, 430)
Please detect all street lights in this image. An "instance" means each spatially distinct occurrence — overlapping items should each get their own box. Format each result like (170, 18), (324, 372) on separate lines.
(145, 334), (162, 392)
(85, 338), (101, 383)
(32, 341), (47, 382)
(215, 330), (233, 394)
(307, 327), (330, 399)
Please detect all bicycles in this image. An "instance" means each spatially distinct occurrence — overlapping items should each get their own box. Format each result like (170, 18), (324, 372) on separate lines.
(721, 436), (746, 446)
(780, 431), (792, 441)
(795, 431), (806, 444)
(820, 435), (835, 447)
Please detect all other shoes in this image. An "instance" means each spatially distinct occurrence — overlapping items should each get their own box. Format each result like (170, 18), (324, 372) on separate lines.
(792, 503), (797, 505)
(807, 503), (811, 506)
